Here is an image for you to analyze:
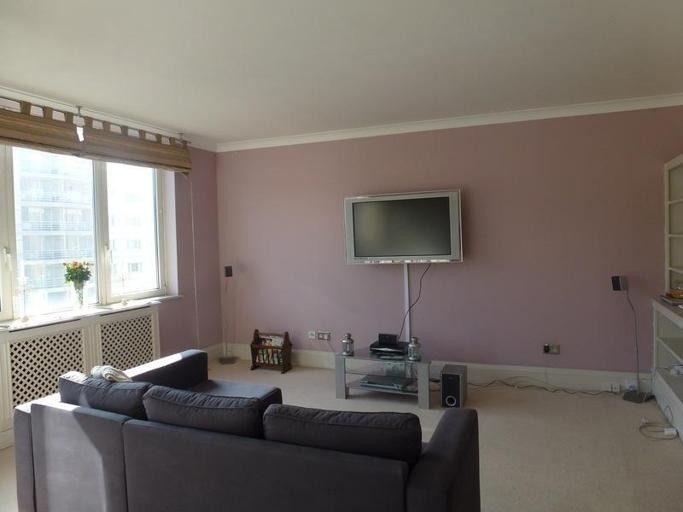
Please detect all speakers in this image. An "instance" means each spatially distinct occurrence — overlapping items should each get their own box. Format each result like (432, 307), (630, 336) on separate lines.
(441, 364), (468, 409)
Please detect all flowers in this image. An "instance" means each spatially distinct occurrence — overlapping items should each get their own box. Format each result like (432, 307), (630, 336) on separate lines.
(63, 260), (92, 283)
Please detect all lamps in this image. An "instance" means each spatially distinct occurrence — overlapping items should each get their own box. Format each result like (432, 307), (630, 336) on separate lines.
(610, 274), (655, 403)
(219, 264), (238, 365)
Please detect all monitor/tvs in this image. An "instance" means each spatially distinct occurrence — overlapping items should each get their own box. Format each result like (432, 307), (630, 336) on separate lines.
(344, 188), (464, 266)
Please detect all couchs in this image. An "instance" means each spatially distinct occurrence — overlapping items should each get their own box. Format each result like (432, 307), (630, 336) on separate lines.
(30, 369), (480, 510)
(13, 349), (282, 510)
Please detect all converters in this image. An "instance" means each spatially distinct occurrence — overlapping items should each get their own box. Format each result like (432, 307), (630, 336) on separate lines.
(663, 427), (677, 436)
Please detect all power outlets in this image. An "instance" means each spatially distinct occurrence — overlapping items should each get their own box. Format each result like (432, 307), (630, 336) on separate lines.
(322, 332), (330, 341)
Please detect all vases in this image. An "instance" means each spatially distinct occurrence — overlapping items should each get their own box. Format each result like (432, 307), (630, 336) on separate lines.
(70, 280), (88, 314)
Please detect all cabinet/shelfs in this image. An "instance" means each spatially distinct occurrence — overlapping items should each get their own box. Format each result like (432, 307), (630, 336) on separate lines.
(649, 298), (682, 440)
(663, 153), (682, 299)
